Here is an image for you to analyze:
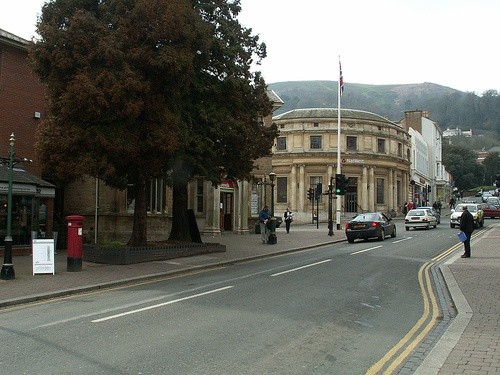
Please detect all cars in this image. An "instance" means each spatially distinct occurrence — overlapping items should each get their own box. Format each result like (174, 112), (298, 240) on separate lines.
(404, 209), (437, 231)
(482, 190), (495, 203)
(344, 212), (395, 243)
(475, 191), (482, 197)
(448, 203), (484, 228)
(486, 197), (500, 205)
(416, 206), (440, 224)
(481, 203), (500, 218)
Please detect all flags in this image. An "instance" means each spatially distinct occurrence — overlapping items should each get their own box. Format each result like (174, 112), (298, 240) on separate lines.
(340, 62), (344, 96)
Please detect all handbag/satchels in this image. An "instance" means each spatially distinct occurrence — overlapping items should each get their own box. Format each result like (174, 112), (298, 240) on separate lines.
(458, 232), (467, 242)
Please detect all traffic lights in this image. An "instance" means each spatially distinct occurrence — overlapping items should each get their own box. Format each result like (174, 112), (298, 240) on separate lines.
(343, 174), (349, 195)
(335, 174), (343, 195)
(306, 188), (312, 201)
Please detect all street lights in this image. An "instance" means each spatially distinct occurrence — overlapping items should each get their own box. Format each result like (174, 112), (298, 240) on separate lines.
(1, 130), (33, 280)
(328, 181), (334, 229)
(269, 172), (277, 244)
(255, 172), (269, 210)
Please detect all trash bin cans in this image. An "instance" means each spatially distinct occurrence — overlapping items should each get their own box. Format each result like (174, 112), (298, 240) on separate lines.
(254, 220), (266, 234)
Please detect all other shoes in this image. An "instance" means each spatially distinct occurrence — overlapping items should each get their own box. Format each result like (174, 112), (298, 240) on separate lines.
(461, 255), (470, 258)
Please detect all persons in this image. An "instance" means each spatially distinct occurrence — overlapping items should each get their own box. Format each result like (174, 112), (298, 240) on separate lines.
(449, 196), (456, 208)
(460, 205), (474, 258)
(259, 206), (270, 243)
(284, 208), (294, 234)
(433, 201), (442, 215)
(474, 205), (484, 230)
(403, 200), (413, 218)
